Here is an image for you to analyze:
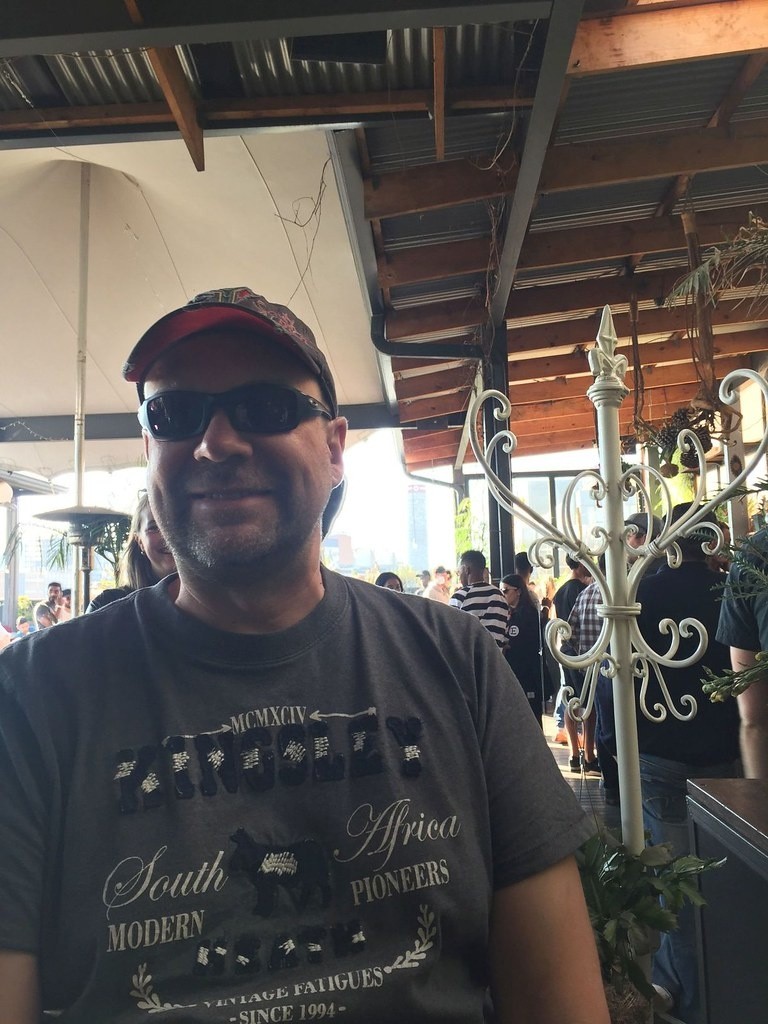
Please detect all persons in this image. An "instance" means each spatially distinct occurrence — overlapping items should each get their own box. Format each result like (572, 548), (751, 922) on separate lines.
(499, 502), (768, 1024)
(450, 550), (510, 653)
(415, 565), (451, 604)
(1, 583), (71, 649)
(375, 573), (402, 592)
(86, 493), (177, 614)
(0, 287), (613, 1023)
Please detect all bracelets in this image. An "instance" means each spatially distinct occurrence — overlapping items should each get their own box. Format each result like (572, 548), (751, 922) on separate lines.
(56, 605), (62, 607)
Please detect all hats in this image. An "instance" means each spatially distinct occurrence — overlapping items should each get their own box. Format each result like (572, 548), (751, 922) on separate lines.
(625, 512), (663, 536)
(416, 570), (430, 577)
(121, 285), (339, 421)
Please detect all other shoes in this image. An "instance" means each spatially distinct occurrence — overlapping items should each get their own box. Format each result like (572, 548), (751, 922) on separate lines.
(583, 758), (602, 776)
(569, 756), (582, 770)
(604, 787), (620, 805)
(555, 732), (569, 746)
(577, 735), (584, 749)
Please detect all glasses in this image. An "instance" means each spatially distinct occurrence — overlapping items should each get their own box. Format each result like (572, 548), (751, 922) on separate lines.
(137, 381), (334, 442)
(501, 587), (519, 593)
